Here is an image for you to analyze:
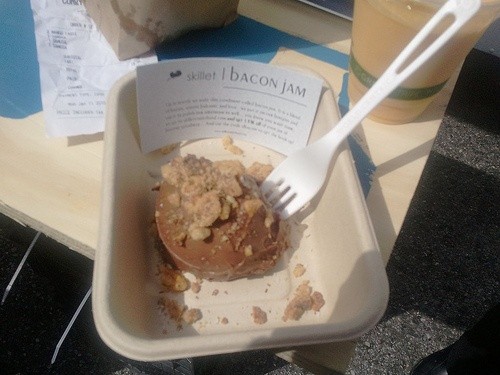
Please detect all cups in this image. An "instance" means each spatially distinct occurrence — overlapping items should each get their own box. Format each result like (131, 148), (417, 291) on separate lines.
(348, 0), (500, 126)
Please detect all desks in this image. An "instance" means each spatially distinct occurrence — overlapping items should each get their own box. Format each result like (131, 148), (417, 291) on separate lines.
(0, 0), (466, 374)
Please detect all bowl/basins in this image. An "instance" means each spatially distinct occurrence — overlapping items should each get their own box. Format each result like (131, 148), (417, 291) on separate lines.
(93, 58), (389, 361)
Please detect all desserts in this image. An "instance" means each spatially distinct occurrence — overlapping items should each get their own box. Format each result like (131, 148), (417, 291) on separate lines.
(152, 152), (282, 293)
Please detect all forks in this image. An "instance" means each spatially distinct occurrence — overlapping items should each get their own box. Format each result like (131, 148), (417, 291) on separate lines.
(259, 0), (482, 220)
